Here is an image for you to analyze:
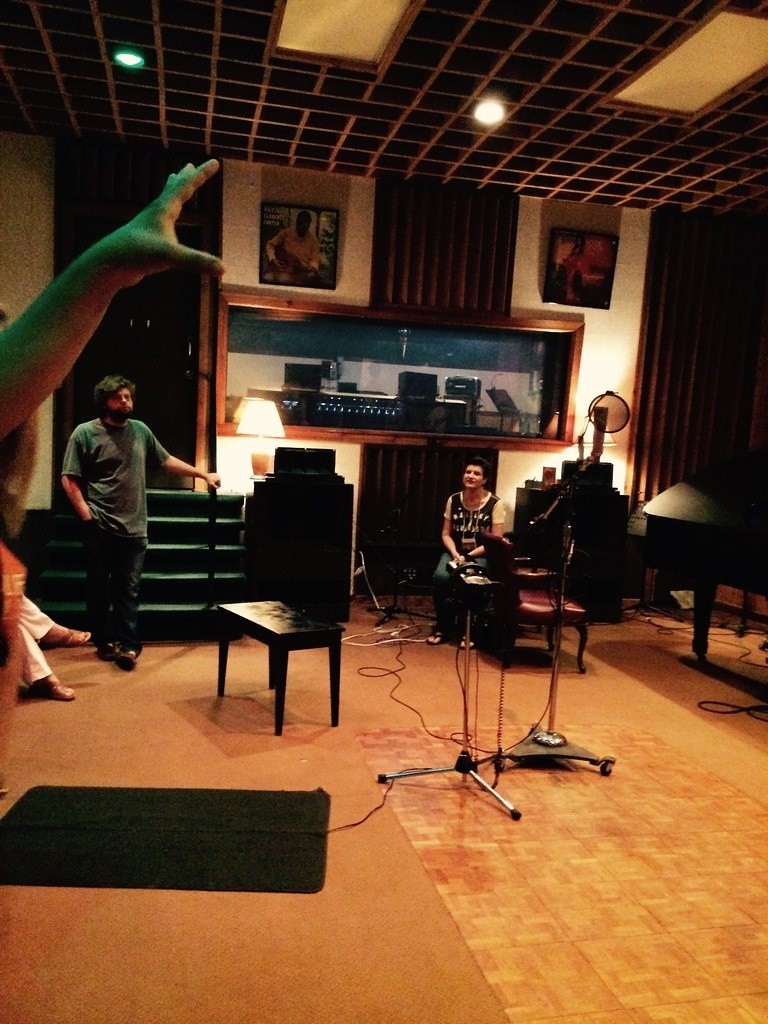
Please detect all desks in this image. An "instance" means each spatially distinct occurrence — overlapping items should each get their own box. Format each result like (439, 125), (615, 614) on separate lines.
(218, 602), (346, 736)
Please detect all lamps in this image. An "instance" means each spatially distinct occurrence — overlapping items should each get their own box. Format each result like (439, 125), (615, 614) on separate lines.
(236, 400), (285, 479)
(233, 398), (264, 422)
(575, 416), (617, 447)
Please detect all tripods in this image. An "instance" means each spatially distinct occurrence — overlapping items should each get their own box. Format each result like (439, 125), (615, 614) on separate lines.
(377, 609), (522, 821)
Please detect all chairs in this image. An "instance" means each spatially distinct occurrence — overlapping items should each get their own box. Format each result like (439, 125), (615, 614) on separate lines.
(478, 532), (588, 673)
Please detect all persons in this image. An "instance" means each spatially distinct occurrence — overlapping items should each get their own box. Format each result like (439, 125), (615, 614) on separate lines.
(0, 160), (228, 438)
(425, 458), (507, 650)
(17, 594), (92, 701)
(61, 377), (222, 672)
(265, 209), (323, 288)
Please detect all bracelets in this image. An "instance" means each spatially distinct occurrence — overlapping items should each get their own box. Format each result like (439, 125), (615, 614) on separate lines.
(463, 553), (474, 562)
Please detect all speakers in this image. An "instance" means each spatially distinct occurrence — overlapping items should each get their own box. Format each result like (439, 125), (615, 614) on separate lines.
(284, 363), (323, 390)
(396, 372), (437, 399)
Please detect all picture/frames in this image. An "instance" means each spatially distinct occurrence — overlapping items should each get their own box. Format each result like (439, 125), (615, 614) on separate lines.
(542, 228), (619, 310)
(259, 202), (340, 290)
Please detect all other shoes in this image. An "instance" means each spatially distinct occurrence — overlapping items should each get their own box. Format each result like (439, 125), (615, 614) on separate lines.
(116, 653), (136, 671)
(96, 643), (116, 660)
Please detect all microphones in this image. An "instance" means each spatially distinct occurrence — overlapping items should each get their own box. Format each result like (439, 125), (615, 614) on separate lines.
(591, 406), (608, 465)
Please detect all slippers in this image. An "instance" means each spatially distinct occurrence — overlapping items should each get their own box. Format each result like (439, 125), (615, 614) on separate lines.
(460, 637), (476, 650)
(426, 635), (446, 644)
(46, 630), (92, 648)
(30, 681), (75, 701)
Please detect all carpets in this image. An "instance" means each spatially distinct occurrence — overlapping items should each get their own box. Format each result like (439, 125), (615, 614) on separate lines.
(0, 786), (330, 893)
